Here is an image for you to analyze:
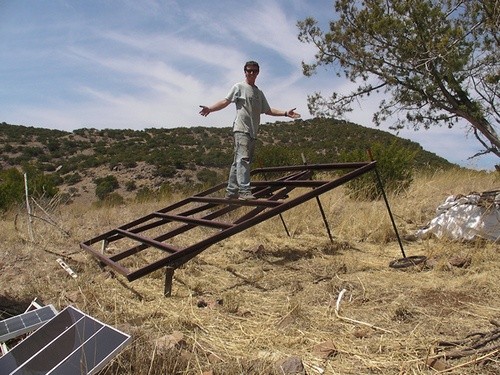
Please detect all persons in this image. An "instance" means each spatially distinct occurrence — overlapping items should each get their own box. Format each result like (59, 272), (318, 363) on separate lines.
(198, 61), (301, 201)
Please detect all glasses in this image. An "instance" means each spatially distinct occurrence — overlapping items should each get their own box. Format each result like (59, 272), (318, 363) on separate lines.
(245, 69), (258, 73)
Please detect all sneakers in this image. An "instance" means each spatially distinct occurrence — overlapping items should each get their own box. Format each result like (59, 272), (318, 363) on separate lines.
(225, 193), (239, 200)
(237, 191), (257, 200)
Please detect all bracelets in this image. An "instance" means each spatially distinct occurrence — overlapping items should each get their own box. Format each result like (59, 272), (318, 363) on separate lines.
(285, 111), (287, 116)
